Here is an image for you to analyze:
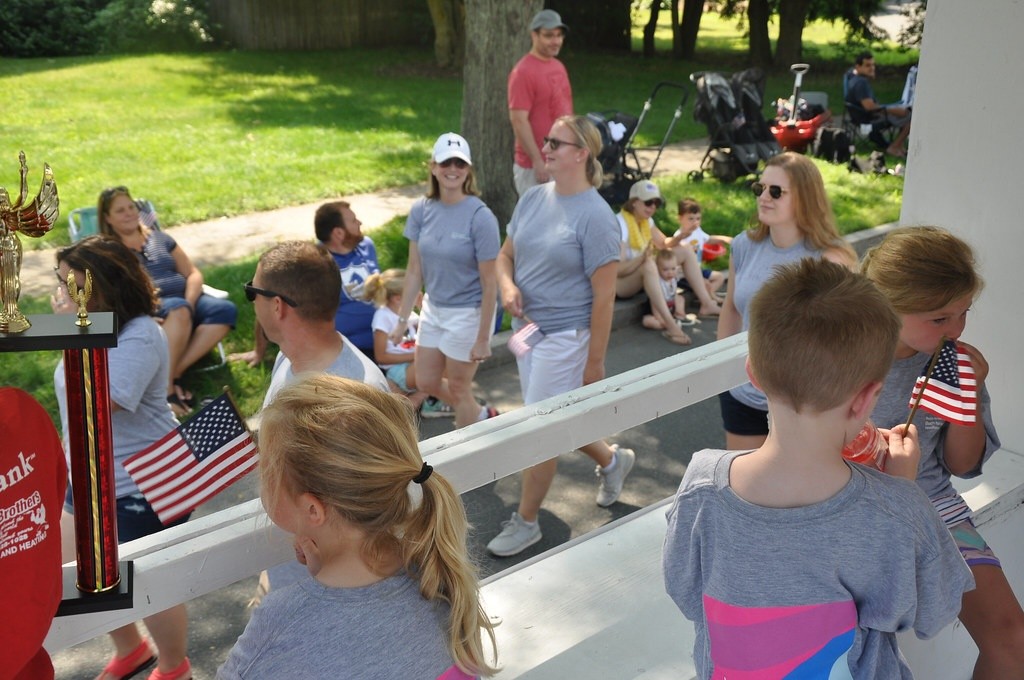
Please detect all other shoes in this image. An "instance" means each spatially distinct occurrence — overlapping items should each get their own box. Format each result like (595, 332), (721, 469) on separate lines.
(697, 310), (719, 319)
(847, 149), (889, 175)
(661, 329), (692, 345)
(673, 315), (696, 327)
(478, 406), (490, 421)
(485, 399), (500, 418)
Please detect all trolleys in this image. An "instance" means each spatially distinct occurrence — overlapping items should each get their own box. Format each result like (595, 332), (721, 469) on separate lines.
(769, 65), (831, 155)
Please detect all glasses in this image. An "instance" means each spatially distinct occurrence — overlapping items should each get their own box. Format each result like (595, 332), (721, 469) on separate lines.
(243, 280), (299, 308)
(645, 199), (662, 208)
(101, 185), (129, 197)
(751, 182), (791, 199)
(54, 265), (84, 295)
(439, 158), (468, 169)
(543, 136), (582, 150)
(538, 29), (566, 39)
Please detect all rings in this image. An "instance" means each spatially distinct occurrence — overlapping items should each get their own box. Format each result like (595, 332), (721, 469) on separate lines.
(56, 299), (64, 306)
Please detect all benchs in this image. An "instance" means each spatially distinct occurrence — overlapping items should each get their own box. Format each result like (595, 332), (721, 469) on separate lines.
(41, 328), (1021, 680)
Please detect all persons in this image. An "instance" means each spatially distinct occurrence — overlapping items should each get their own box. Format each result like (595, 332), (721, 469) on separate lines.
(663, 256), (977, 680)
(857, 227), (1024, 680)
(225, 202), (486, 418)
(98, 186), (238, 417)
(508, 10), (573, 198)
(390, 131), (501, 429)
(50, 236), (196, 680)
(716, 152), (860, 451)
(212, 373), (503, 679)
(614, 180), (732, 345)
(242, 240), (391, 411)
(487, 115), (635, 556)
(0, 386), (69, 679)
(844, 51), (911, 159)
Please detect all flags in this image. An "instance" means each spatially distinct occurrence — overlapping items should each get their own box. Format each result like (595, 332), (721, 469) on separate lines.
(122, 392), (260, 527)
(909, 336), (977, 428)
(505, 323), (545, 357)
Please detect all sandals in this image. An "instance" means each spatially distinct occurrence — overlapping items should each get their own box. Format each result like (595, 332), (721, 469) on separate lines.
(92, 637), (159, 680)
(146, 656), (194, 680)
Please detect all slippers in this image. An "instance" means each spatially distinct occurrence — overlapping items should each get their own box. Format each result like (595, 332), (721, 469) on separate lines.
(167, 392), (194, 417)
(173, 377), (196, 407)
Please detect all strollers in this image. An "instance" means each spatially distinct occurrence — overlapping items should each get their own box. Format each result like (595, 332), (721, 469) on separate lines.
(687, 68), (782, 188)
(588, 80), (689, 214)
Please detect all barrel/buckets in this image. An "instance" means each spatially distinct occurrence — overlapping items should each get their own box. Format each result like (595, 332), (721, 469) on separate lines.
(702, 242), (726, 261)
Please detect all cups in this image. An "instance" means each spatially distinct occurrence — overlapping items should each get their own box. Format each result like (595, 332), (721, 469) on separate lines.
(840, 417), (890, 471)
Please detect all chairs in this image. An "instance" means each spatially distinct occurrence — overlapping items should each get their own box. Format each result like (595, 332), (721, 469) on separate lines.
(840, 70), (895, 149)
(69, 199), (228, 374)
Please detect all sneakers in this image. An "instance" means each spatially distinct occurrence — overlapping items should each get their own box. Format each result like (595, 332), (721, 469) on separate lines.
(595, 443), (636, 508)
(487, 511), (542, 557)
(420, 399), (457, 418)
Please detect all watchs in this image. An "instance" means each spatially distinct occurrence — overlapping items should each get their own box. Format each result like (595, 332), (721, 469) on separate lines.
(398, 316), (408, 324)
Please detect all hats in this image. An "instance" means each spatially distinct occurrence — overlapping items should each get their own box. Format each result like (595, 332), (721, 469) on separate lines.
(531, 9), (571, 33)
(431, 131), (472, 165)
(629, 180), (661, 201)
